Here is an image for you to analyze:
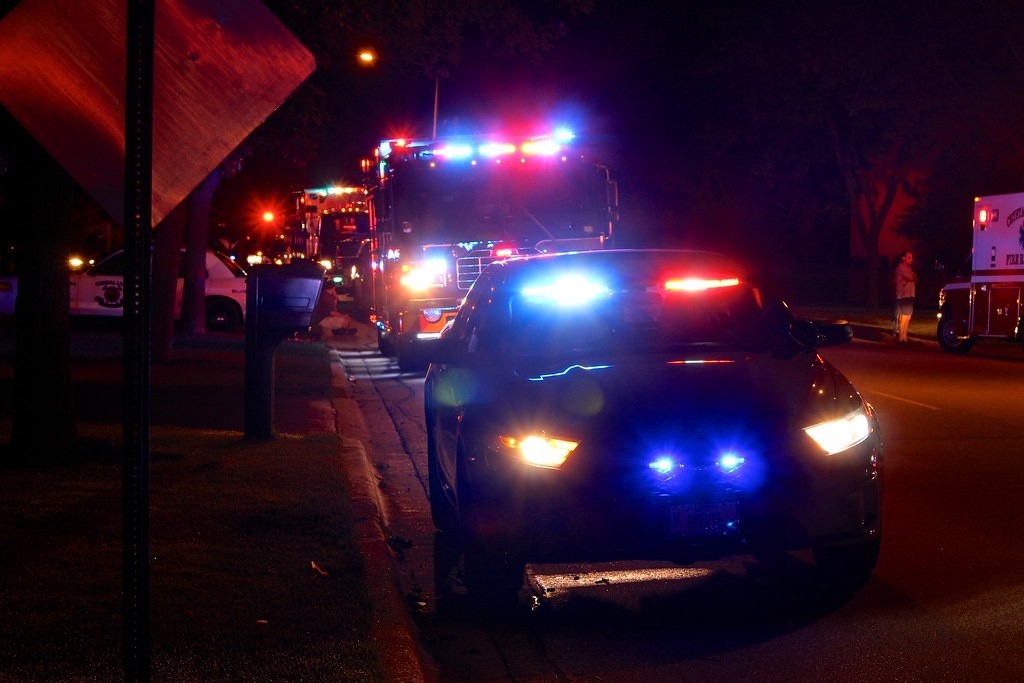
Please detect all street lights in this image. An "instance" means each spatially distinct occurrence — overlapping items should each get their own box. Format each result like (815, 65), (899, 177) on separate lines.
(358, 47), (440, 141)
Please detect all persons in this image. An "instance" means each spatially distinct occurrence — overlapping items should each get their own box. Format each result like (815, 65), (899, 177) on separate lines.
(895, 251), (918, 344)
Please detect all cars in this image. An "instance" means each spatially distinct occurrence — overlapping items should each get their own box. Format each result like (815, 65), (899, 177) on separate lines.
(68, 243), (248, 332)
(398, 247), (884, 586)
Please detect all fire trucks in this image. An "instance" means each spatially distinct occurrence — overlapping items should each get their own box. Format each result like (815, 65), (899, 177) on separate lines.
(935, 193), (1024, 359)
(361, 130), (621, 373)
(291, 186), (366, 295)
